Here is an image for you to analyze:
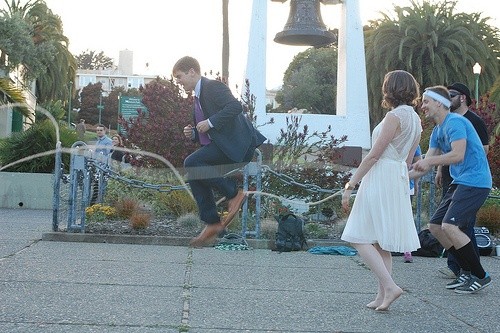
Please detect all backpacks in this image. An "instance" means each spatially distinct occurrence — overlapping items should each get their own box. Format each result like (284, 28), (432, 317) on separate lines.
(272, 212), (307, 252)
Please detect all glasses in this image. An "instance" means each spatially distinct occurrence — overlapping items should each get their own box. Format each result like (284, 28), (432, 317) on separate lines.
(450, 92), (463, 97)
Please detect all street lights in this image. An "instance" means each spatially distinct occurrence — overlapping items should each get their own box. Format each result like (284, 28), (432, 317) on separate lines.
(473, 61), (481, 113)
(118, 91), (122, 134)
(99, 90), (102, 126)
(69, 80), (72, 128)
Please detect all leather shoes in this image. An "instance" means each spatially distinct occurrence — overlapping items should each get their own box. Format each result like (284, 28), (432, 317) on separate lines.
(188, 222), (223, 244)
(224, 190), (249, 227)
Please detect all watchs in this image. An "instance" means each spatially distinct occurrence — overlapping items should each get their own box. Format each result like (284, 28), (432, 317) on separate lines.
(344, 182), (354, 191)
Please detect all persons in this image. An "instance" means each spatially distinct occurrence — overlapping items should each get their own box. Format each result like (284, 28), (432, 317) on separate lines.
(108, 135), (124, 165)
(89, 124), (112, 206)
(424, 84), (490, 285)
(403, 139), (426, 263)
(170, 56), (266, 248)
(76, 119), (86, 141)
(405, 84), (493, 294)
(343, 69), (423, 313)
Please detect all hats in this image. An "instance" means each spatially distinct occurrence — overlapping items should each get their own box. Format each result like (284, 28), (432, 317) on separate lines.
(446, 82), (472, 106)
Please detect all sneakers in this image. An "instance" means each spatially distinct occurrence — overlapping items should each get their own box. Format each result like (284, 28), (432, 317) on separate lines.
(454, 273), (492, 294)
(445, 273), (471, 289)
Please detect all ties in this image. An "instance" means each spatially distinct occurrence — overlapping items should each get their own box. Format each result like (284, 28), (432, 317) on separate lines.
(193, 94), (210, 145)
